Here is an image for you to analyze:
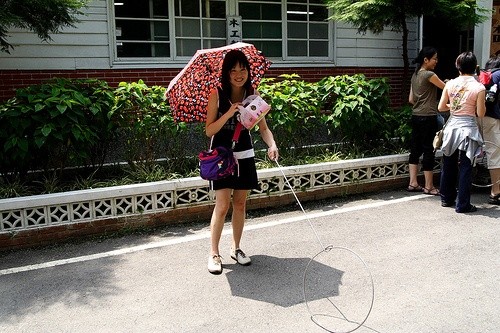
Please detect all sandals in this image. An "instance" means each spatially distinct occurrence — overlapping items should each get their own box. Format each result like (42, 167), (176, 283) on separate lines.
(423, 186), (440, 195)
(487, 192), (500, 204)
(406, 184), (424, 192)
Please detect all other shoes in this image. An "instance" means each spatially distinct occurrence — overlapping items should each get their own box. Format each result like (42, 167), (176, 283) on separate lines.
(442, 201), (457, 207)
(455, 204), (477, 212)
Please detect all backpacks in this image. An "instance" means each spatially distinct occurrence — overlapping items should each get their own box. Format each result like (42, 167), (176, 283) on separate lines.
(476, 67), (500, 102)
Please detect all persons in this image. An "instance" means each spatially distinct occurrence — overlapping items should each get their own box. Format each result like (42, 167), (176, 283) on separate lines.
(408, 47), (500, 214)
(205, 52), (279, 273)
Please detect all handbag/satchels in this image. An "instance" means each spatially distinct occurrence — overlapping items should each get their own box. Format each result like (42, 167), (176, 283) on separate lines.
(432, 129), (443, 149)
(198, 146), (234, 180)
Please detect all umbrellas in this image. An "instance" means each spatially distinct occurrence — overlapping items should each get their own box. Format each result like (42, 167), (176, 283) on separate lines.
(166, 43), (272, 124)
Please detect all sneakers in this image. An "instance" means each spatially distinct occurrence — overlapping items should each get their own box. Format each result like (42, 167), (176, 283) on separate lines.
(229, 247), (252, 266)
(207, 254), (225, 274)
